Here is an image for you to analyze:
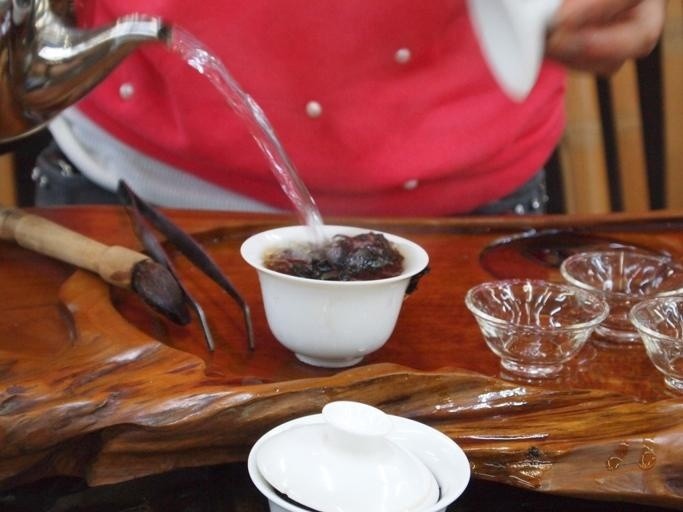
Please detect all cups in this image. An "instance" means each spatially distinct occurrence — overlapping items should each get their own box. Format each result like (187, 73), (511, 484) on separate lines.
(561, 250), (683, 344)
(248, 399), (472, 512)
(627, 293), (683, 393)
(465, 278), (611, 377)
(238, 224), (430, 370)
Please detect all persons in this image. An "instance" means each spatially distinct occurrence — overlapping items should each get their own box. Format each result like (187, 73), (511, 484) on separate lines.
(30, 1), (664, 216)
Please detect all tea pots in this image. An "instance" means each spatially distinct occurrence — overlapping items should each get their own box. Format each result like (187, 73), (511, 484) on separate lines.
(0, 0), (174, 147)
